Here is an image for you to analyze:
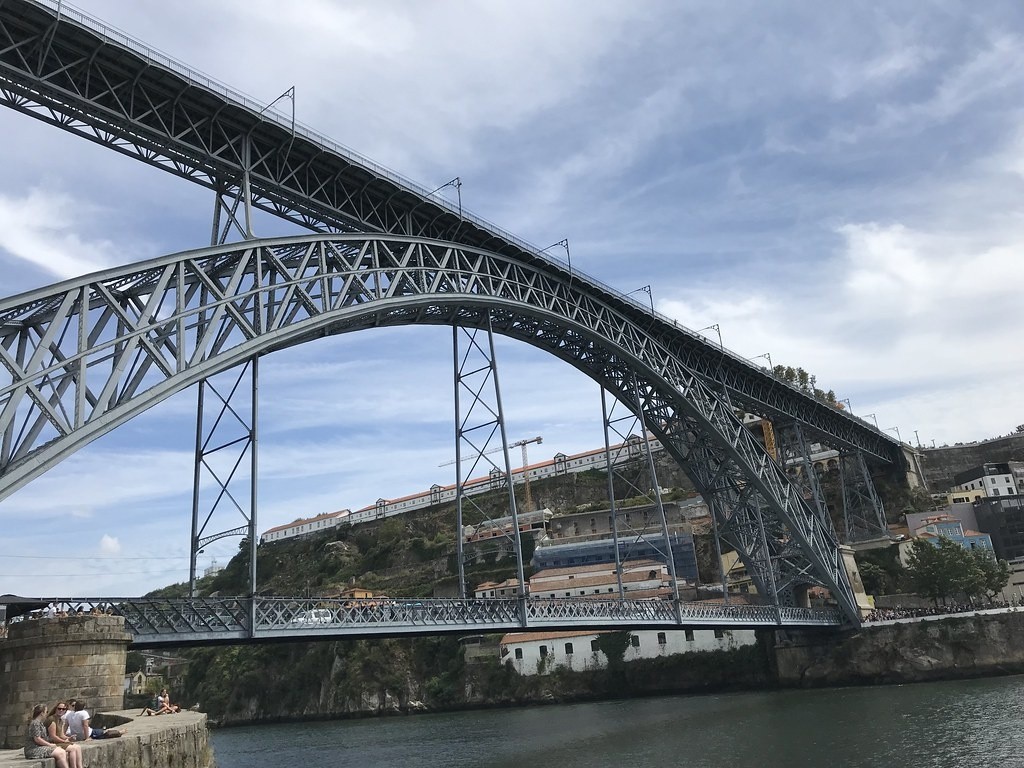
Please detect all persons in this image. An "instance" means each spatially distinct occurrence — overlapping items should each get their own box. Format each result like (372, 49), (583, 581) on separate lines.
(333, 600), (399, 624)
(24, 704), (69, 768)
(864, 596), (1024, 623)
(61, 699), (128, 742)
(32, 602), (113, 620)
(43, 702), (83, 768)
(136, 689), (181, 716)
(0, 617), (9, 638)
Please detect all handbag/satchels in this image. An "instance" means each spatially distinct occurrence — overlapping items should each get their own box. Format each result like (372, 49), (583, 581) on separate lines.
(104, 730), (121, 738)
(90, 729), (106, 739)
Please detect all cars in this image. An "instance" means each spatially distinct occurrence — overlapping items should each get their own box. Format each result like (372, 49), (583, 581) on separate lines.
(288, 609), (332, 625)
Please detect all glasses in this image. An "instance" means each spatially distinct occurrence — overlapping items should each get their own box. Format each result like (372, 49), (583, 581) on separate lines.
(70, 704), (75, 707)
(57, 706), (67, 711)
(46, 711), (48, 713)
